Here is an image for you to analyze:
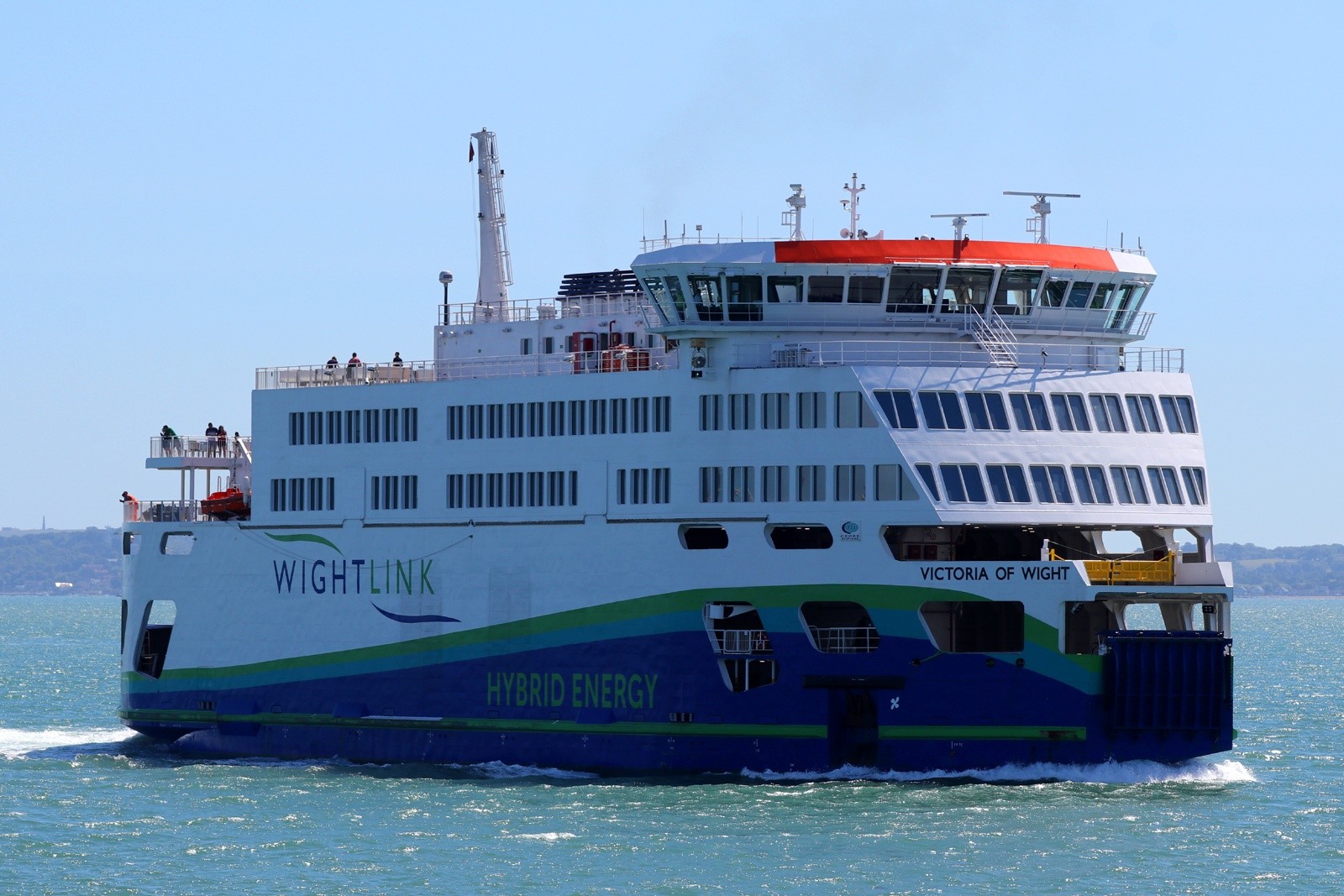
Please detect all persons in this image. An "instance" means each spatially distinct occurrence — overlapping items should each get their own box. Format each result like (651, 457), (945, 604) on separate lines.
(234, 432), (242, 459)
(326, 357), (339, 386)
(348, 353), (361, 367)
(393, 352), (402, 383)
(160, 425), (181, 457)
(216, 426), (227, 458)
(119, 491), (138, 521)
(693, 289), (703, 319)
(206, 423), (218, 458)
(717, 289), (730, 321)
(673, 289), (687, 322)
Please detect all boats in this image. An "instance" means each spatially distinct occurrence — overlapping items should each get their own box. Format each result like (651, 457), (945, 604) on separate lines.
(117, 132), (1238, 787)
(200, 488), (251, 515)
